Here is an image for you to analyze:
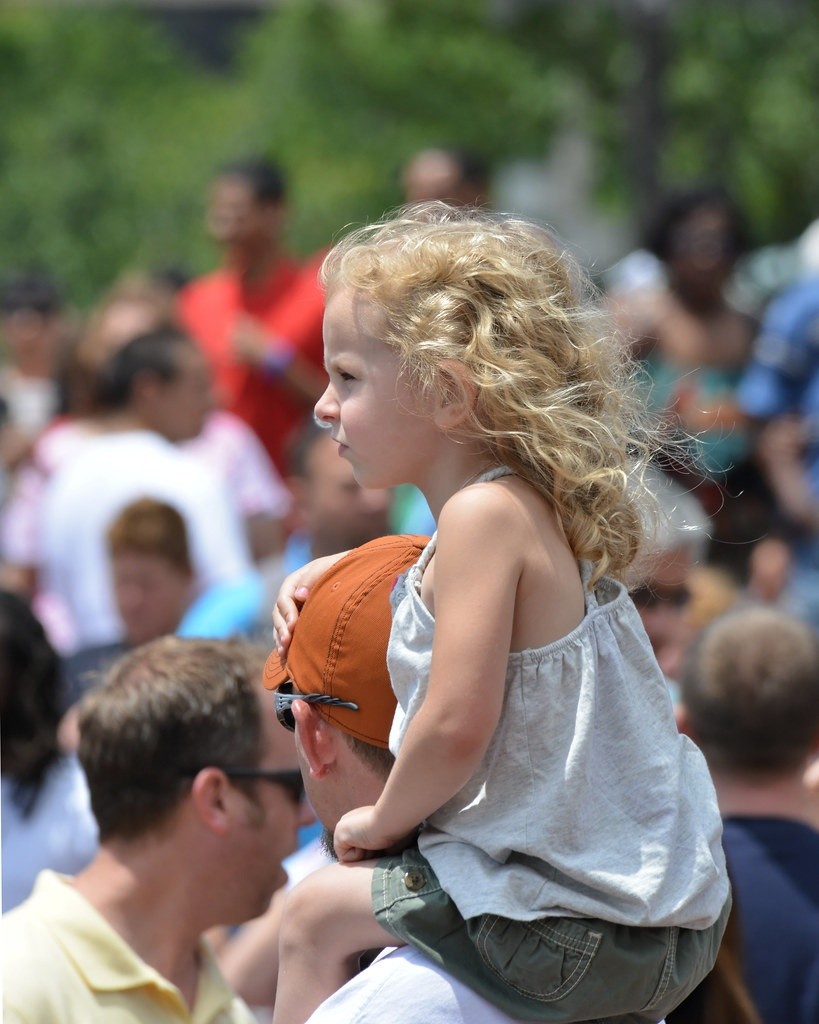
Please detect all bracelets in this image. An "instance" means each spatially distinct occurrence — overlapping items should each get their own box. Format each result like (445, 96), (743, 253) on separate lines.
(269, 348), (292, 375)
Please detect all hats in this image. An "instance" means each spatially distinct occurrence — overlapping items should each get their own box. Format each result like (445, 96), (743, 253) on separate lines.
(262, 533), (434, 750)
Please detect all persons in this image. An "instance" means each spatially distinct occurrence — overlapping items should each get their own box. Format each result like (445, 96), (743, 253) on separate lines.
(672, 603), (819, 1024)
(0, 591), (100, 914)
(0, 146), (819, 756)
(0, 635), (317, 1024)
(272, 199), (733, 1024)
(263, 534), (664, 1024)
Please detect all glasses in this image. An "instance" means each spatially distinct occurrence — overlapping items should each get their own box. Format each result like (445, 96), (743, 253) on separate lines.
(173, 761), (308, 805)
(272, 686), (359, 732)
(630, 577), (693, 609)
